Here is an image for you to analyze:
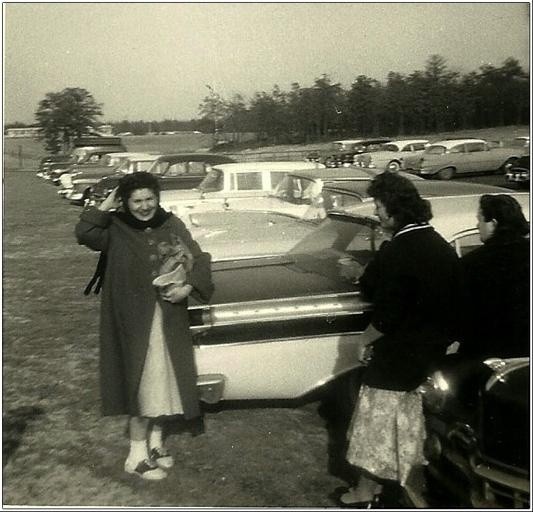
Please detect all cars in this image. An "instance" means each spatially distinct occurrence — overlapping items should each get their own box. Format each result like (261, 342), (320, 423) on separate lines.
(34, 129), (516, 267)
(393, 353), (530, 510)
(504, 135), (531, 192)
(175, 192), (530, 416)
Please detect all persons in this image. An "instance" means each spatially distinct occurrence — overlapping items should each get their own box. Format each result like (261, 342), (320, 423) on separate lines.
(328, 172), (461, 509)
(75, 171), (215, 482)
(358, 193), (529, 393)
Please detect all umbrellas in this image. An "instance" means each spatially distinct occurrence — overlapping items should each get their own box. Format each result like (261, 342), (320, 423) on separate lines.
(334, 486), (383, 508)
(327, 492), (373, 508)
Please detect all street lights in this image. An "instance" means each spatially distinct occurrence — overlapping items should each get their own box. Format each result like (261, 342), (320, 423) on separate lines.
(151, 232), (193, 293)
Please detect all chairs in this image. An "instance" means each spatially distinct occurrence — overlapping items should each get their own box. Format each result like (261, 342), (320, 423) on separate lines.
(150, 447), (174, 468)
(124, 459), (167, 480)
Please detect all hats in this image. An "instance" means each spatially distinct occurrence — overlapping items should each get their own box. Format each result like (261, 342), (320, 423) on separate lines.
(393, 353), (530, 510)
(175, 192), (530, 416)
